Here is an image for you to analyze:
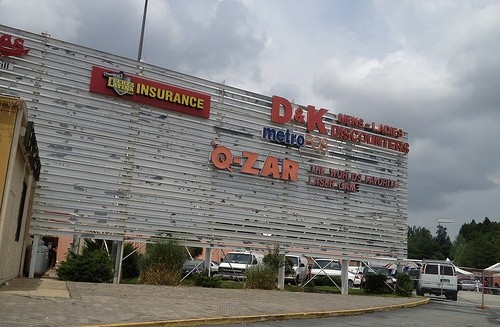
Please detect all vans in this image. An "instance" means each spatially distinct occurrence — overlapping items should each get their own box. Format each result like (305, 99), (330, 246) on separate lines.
(311, 259), (343, 285)
(416, 261), (458, 302)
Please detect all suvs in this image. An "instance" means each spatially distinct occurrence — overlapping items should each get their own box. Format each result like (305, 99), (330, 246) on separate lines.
(218, 252), (258, 281)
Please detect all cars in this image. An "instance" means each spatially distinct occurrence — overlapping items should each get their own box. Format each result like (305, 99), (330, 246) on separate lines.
(181, 259), (220, 277)
(348, 265), (399, 294)
(457, 279), (484, 291)
(284, 254), (309, 285)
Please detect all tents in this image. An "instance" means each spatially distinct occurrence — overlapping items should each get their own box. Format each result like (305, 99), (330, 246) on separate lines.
(445, 258), (473, 281)
(481, 262), (500, 309)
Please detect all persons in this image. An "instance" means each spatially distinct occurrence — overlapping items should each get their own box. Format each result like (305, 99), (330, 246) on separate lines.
(307, 265), (313, 281)
(475, 277), (500, 296)
(395, 261), (411, 277)
(46, 239), (57, 269)
